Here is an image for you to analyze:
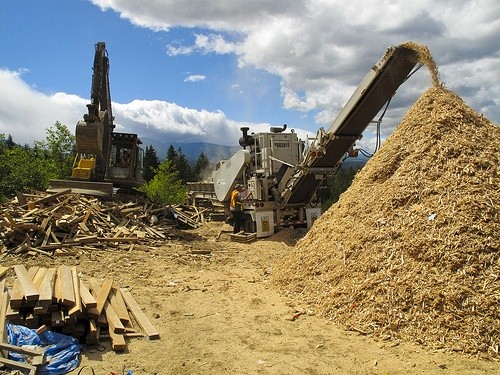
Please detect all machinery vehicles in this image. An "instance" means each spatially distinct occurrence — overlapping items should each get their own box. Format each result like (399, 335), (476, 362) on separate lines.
(211, 43), (421, 240)
(71, 40), (148, 197)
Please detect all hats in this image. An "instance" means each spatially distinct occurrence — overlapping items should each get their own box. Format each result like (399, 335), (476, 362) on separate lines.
(235, 184), (243, 189)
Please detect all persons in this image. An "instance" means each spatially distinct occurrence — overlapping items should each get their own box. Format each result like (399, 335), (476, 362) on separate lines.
(229, 184), (253, 234)
(120, 151), (131, 166)
